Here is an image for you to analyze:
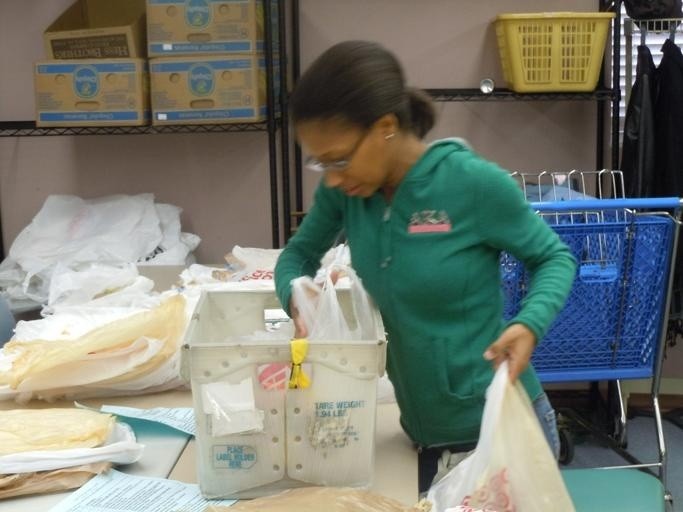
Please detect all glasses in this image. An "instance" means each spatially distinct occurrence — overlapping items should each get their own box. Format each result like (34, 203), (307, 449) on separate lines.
(300, 123), (376, 174)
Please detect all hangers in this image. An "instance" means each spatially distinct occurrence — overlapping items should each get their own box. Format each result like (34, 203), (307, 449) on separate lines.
(637, 24), (678, 47)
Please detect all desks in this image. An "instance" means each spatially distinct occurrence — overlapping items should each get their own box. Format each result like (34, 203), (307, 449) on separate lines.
(0, 377), (419, 512)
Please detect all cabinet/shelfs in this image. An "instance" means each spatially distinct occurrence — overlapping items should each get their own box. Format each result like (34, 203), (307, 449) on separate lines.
(269, 0), (620, 253)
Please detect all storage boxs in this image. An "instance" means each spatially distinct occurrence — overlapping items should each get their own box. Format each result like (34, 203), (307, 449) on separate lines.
(181, 290), (387, 501)
(34, 0), (267, 129)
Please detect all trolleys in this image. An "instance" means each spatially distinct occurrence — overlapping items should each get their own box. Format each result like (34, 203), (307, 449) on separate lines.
(500, 168), (683, 512)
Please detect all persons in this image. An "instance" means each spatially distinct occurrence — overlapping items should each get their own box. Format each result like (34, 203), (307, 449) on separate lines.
(272, 37), (581, 497)
